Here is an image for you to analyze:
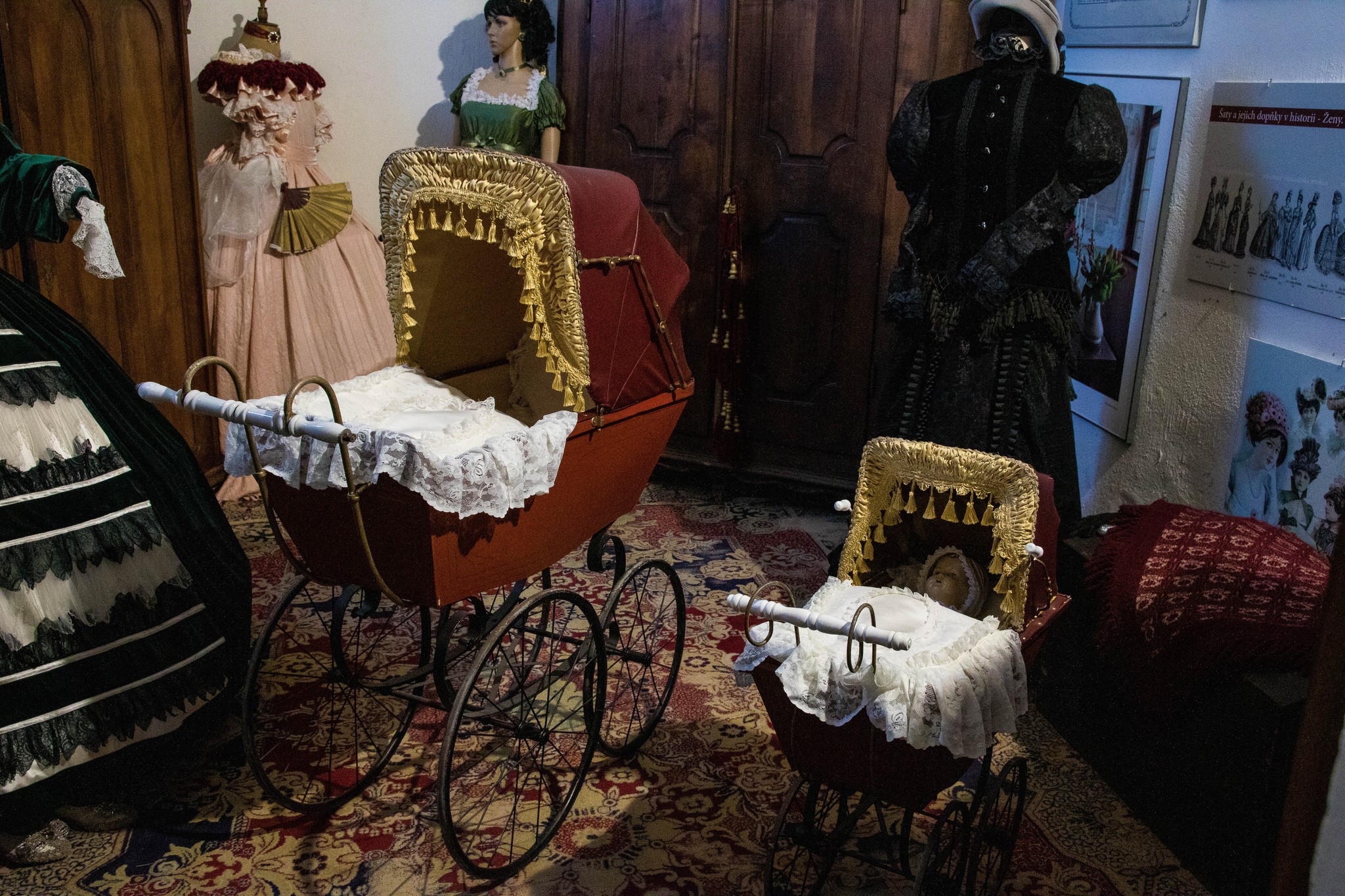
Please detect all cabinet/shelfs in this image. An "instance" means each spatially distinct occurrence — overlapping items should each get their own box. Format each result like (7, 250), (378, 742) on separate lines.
(556, 0), (981, 505)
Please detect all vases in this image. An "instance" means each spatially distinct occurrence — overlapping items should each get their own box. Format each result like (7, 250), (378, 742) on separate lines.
(1086, 301), (1103, 344)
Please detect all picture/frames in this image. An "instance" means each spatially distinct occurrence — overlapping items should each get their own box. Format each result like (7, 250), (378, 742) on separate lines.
(1057, 72), (1190, 442)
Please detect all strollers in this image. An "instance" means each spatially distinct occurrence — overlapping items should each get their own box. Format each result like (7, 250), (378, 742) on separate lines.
(131, 146), (695, 876)
(725, 439), (1075, 896)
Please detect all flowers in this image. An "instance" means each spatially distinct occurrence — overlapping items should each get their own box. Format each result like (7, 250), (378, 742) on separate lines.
(1072, 217), (1127, 302)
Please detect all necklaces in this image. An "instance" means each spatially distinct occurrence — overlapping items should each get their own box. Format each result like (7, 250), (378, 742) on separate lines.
(1245, 464), (1262, 497)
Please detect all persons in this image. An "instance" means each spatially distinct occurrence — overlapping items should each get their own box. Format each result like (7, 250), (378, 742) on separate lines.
(917, 545), (990, 619)
(191, 0), (395, 404)
(884, 0), (1132, 548)
(0, 126), (254, 863)
(1226, 377), (1345, 561)
(448, 2), (571, 171)
